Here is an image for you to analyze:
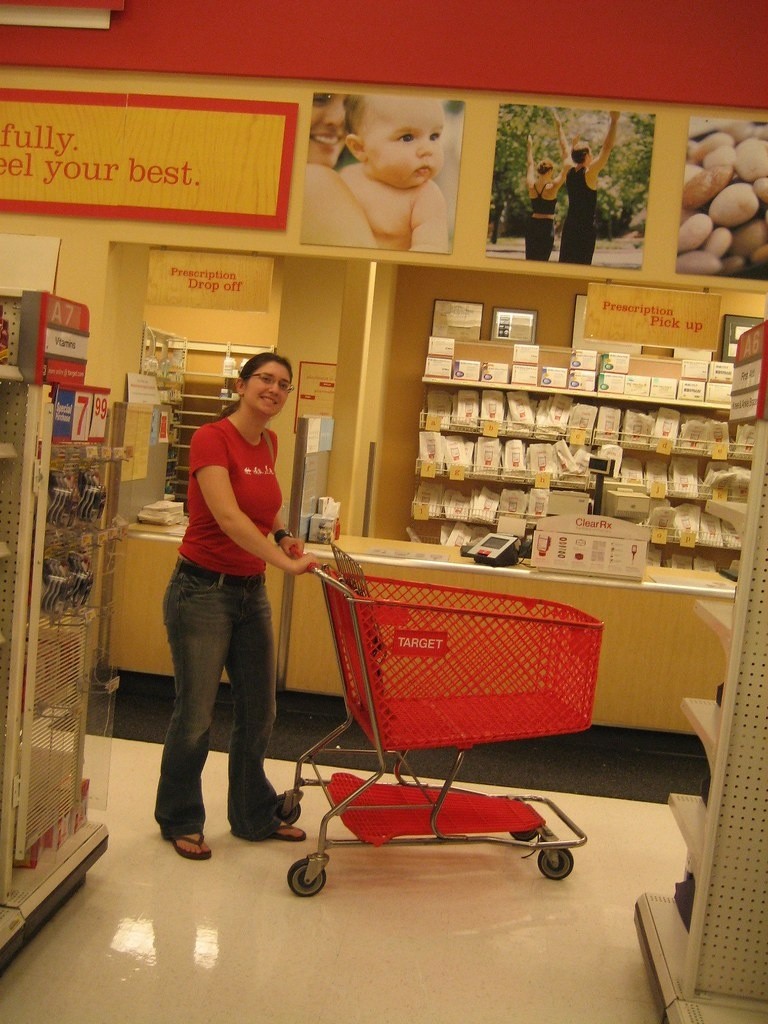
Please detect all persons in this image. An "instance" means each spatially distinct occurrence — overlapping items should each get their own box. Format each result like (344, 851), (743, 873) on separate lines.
(551, 107), (620, 265)
(301, 92), (379, 249)
(337, 94), (450, 255)
(155, 352), (324, 861)
(524, 132), (582, 262)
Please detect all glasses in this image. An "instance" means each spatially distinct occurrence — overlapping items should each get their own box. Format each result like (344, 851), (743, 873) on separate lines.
(240, 372), (295, 393)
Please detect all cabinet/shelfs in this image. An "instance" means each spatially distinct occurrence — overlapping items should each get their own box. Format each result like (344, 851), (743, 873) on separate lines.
(139, 322), (275, 516)
(0, 288), (129, 977)
(636, 421), (768, 1023)
(410, 342), (755, 571)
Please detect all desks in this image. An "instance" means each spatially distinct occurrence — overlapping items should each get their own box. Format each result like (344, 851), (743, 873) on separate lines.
(109, 403), (736, 736)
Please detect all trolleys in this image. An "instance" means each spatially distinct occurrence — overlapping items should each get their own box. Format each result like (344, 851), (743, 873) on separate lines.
(276, 544), (605, 896)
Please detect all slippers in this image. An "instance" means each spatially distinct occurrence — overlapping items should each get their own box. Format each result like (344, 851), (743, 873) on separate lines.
(267, 823), (306, 842)
(170, 834), (211, 860)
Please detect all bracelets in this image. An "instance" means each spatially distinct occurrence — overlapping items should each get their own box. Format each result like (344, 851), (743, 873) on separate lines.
(274, 528), (294, 545)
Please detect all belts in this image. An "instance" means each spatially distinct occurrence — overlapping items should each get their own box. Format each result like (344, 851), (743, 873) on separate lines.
(175, 556), (266, 588)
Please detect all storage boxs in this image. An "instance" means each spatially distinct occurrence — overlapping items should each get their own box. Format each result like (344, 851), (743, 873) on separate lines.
(425, 336), (735, 403)
